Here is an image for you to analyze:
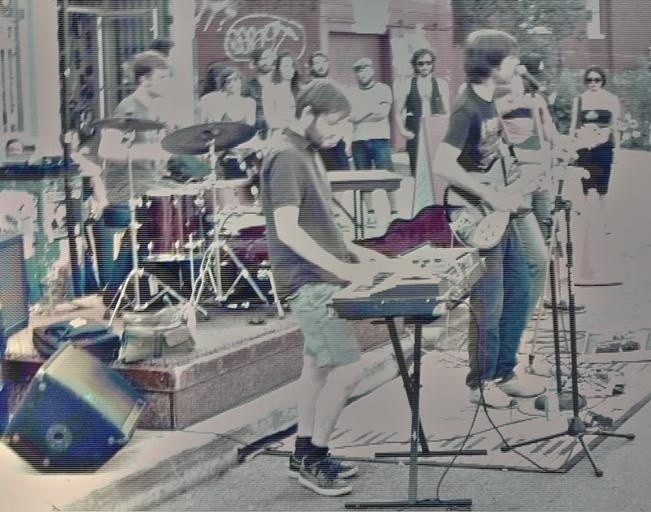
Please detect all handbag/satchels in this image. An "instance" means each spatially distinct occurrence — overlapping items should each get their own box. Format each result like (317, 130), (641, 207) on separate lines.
(33, 316), (120, 363)
(119, 309), (195, 363)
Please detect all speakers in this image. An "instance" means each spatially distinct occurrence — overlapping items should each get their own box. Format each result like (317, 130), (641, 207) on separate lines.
(1, 341), (147, 473)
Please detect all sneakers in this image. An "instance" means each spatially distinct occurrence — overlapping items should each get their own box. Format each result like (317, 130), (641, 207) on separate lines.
(289, 450), (358, 497)
(468, 364), (548, 409)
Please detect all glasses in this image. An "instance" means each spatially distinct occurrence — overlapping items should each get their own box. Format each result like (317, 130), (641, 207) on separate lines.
(585, 77), (602, 83)
(416, 61), (431, 66)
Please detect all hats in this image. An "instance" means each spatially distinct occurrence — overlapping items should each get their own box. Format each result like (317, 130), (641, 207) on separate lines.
(136, 49), (172, 70)
(354, 57), (372, 67)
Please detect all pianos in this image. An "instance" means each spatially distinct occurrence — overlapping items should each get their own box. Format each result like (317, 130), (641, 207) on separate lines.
(326, 244), (488, 320)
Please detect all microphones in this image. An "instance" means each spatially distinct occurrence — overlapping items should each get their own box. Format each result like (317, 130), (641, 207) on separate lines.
(515, 64), (550, 96)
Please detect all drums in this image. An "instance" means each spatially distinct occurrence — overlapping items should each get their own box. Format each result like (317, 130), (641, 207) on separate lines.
(188, 177), (262, 224)
(204, 214), (275, 310)
(135, 189), (207, 263)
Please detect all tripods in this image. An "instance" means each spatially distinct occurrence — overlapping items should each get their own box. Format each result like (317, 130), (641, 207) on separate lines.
(501, 106), (635, 477)
(103, 145), (210, 331)
(189, 138), (269, 319)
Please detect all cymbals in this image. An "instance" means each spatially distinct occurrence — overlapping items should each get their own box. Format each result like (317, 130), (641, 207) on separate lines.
(90, 116), (165, 131)
(161, 122), (255, 155)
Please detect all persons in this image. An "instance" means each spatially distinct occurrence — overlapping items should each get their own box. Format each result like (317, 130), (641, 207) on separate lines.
(3, 47), (623, 356)
(432, 29), (581, 411)
(260, 77), (393, 499)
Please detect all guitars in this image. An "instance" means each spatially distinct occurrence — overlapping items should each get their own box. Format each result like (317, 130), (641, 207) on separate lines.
(444, 123), (600, 252)
